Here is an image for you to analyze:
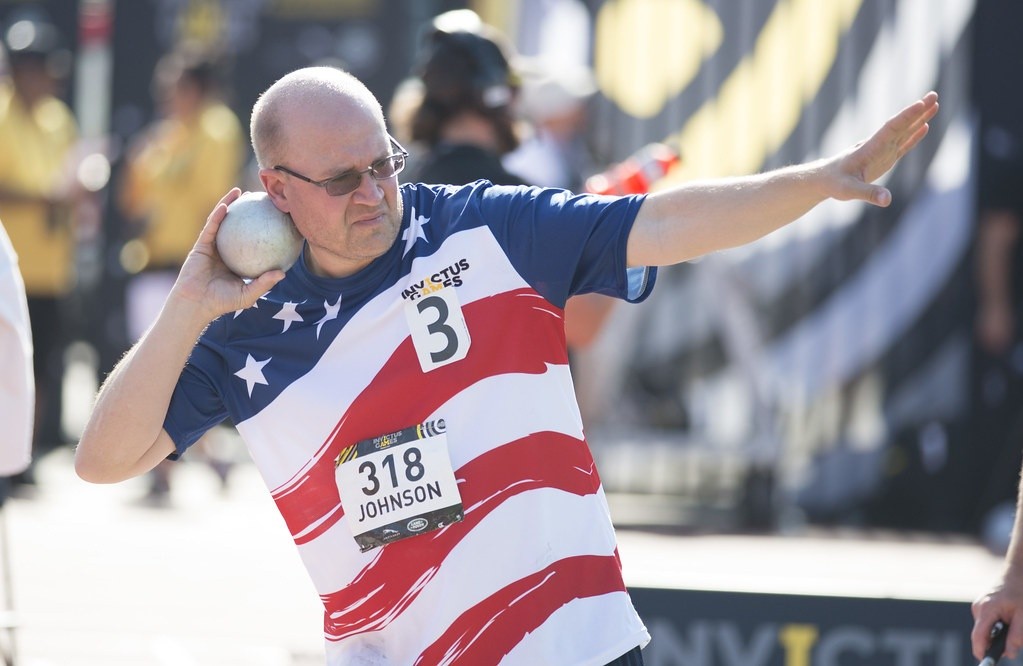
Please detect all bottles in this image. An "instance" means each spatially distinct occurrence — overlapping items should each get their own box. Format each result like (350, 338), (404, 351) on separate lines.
(587, 145), (679, 196)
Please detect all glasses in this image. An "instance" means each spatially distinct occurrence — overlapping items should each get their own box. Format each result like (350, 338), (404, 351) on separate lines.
(274, 135), (410, 196)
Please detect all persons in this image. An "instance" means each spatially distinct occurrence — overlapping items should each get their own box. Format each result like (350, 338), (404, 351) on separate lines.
(0, 223), (37, 665)
(952, 1), (1022, 533)
(73, 65), (939, 666)
(118, 57), (247, 514)
(1, 18), (84, 460)
(386, 34), (615, 382)
(970, 470), (1023, 664)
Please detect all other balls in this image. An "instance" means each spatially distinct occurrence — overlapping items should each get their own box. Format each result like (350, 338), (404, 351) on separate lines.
(215, 190), (305, 281)
